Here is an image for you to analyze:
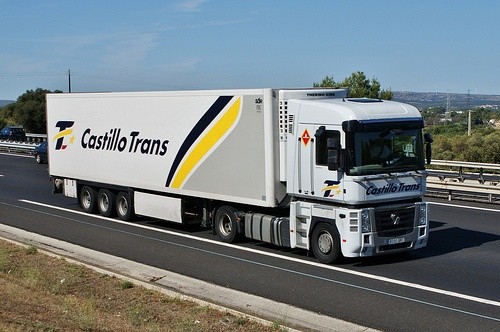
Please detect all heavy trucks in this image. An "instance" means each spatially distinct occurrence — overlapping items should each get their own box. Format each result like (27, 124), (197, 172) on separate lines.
(42, 87), (431, 264)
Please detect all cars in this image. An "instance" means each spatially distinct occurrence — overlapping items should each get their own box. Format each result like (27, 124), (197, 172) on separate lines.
(2, 126), (25, 140)
(34, 140), (48, 163)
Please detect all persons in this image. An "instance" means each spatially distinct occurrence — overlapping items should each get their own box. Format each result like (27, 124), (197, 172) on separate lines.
(369, 133), (404, 164)
